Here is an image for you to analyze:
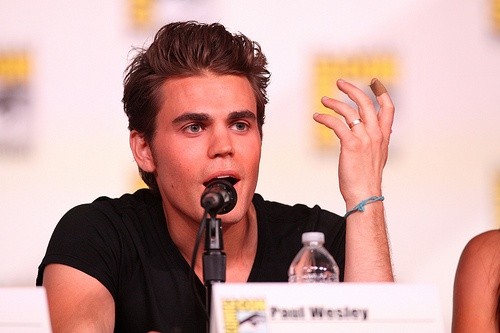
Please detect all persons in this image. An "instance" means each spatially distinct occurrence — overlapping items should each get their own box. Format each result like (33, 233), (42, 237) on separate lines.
(34, 19), (395, 333)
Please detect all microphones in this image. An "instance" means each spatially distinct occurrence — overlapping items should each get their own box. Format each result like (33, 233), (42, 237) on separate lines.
(199, 179), (238, 216)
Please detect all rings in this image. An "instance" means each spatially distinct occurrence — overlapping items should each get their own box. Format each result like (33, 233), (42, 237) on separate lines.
(348, 119), (363, 128)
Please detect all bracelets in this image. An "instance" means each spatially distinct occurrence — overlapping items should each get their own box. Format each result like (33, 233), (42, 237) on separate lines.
(344, 196), (385, 219)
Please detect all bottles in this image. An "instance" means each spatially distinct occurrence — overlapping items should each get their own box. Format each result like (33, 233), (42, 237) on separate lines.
(288, 232), (339, 283)
